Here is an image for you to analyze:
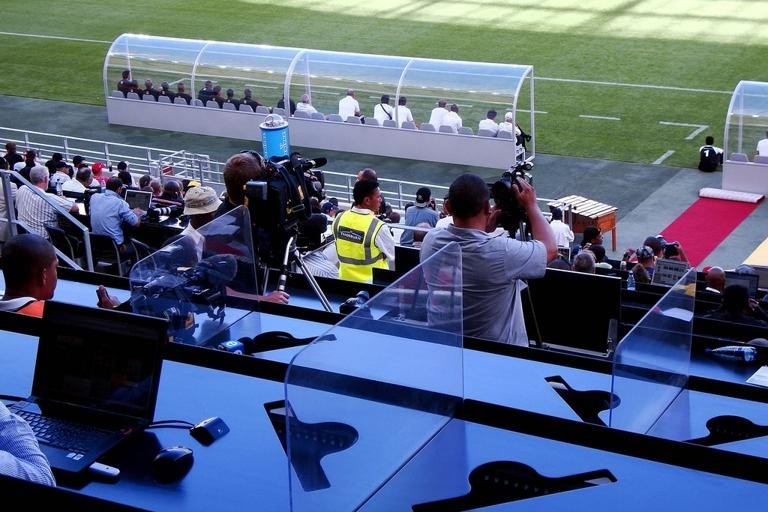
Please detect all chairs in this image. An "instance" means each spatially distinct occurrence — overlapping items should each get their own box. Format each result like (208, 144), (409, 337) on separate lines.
(370, 245), (768, 364)
(256, 107), (269, 115)
(294, 112), (310, 119)
(311, 114), (324, 121)
(458, 129), (473, 136)
(89, 236), (132, 278)
(383, 121), (396, 128)
(190, 100), (203, 107)
(497, 132), (526, 163)
(239, 105), (254, 113)
(112, 92), (124, 98)
(127, 94), (139, 100)
(753, 156), (768, 165)
(347, 117), (360, 125)
(206, 102), (219, 109)
(223, 104), (236, 111)
(273, 109), (285, 115)
(327, 116), (343, 123)
(174, 98), (187, 105)
(365, 120), (378, 126)
(158, 97), (171, 104)
(401, 123), (415, 130)
(730, 154), (748, 163)
(478, 130), (493, 138)
(422, 125), (435, 132)
(43, 224), (85, 268)
(439, 127), (452, 134)
(142, 95), (155, 102)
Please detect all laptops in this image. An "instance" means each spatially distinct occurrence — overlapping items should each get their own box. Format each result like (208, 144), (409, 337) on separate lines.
(124, 189), (153, 216)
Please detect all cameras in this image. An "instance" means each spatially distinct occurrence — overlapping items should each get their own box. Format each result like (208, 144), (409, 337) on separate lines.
(246, 154), (327, 269)
(130, 253), (238, 341)
(339, 290), (373, 319)
(491, 161), (535, 238)
(146, 205), (180, 218)
(664, 243), (679, 259)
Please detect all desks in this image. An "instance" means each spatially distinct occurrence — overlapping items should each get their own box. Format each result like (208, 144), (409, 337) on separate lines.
(547, 195), (619, 253)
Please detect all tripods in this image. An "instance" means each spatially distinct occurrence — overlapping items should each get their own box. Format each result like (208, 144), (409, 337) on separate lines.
(261, 237), (335, 312)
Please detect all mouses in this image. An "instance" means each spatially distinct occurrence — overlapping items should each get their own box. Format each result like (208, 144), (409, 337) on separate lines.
(154, 446), (193, 479)
(140, 214), (146, 220)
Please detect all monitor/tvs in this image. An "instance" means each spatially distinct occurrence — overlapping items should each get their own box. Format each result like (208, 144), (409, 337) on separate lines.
(754, 267), (768, 288)
(722, 271), (759, 297)
(520, 268), (622, 357)
(651, 259), (688, 288)
(558, 247), (570, 263)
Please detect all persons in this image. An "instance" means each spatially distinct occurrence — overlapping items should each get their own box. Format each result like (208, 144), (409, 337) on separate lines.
(173, 83), (193, 107)
(546, 208), (574, 262)
(14, 166), (83, 270)
(203, 148), (290, 306)
(549, 253), (593, 275)
(443, 104), (461, 134)
(420, 172), (557, 347)
(401, 189), (439, 247)
(297, 93), (318, 118)
(73, 156), (85, 176)
(333, 180), (380, 287)
(3, 142), (21, 166)
(734, 264), (759, 295)
(499, 113), (529, 151)
(478, 111), (499, 135)
(158, 82), (175, 103)
(239, 89), (260, 112)
(393, 96), (417, 131)
(223, 87), (239, 111)
(92, 163), (109, 188)
(374, 93), (393, 128)
(142, 79), (155, 100)
(0, 158), (18, 246)
(152, 167), (181, 190)
(352, 170), (377, 184)
(181, 180), (203, 196)
(206, 87), (221, 110)
(430, 99), (449, 131)
(708, 284), (753, 325)
(378, 200), (414, 227)
(44, 153), (65, 175)
(339, 89), (365, 124)
(89, 176), (145, 262)
(197, 82), (212, 105)
(13, 150), (41, 171)
(115, 163), (134, 189)
(645, 236), (691, 273)
(128, 79), (141, 98)
(168, 186), (224, 261)
(580, 227), (602, 254)
(151, 180), (188, 209)
(588, 244), (610, 272)
(278, 90), (297, 115)
(621, 247), (657, 281)
(409, 223), (433, 251)
(319, 198), (338, 235)
(1, 235), (121, 320)
(118, 70), (134, 96)
(704, 264), (719, 291)
(422, 196), (451, 220)
(119, 171), (163, 197)
(754, 131), (768, 163)
(696, 135), (723, 172)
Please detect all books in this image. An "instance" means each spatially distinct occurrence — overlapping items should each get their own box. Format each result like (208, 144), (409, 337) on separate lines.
(120, 190), (152, 222)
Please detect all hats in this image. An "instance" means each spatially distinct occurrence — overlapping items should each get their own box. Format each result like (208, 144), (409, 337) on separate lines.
(583, 226), (602, 242)
(92, 162), (102, 174)
(637, 245), (655, 258)
(415, 187), (431, 208)
(505, 112), (513, 120)
(183, 184), (222, 216)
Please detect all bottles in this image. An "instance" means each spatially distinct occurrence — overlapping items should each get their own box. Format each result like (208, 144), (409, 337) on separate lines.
(56, 178), (63, 197)
(101, 180), (106, 193)
(627, 270), (635, 291)
(578, 246), (582, 253)
(108, 160), (112, 172)
(704, 345), (757, 362)
